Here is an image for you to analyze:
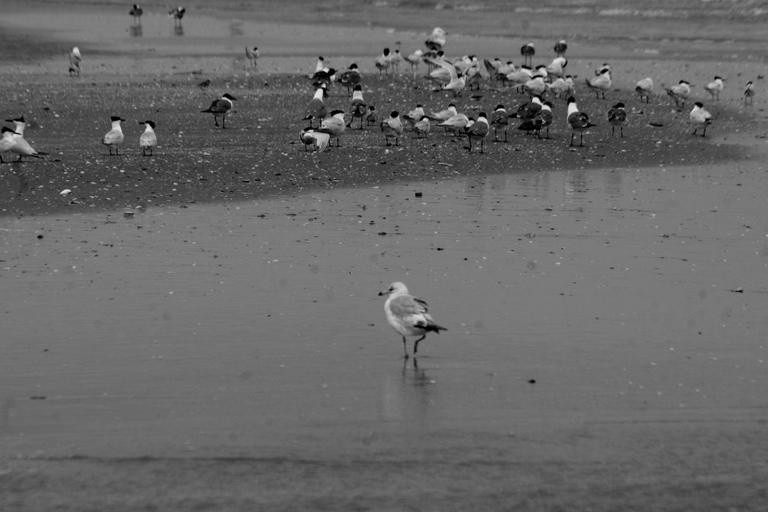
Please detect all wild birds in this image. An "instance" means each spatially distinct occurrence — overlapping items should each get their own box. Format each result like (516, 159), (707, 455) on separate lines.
(4, 116), (49, 163)
(689, 101), (714, 137)
(245, 47), (259, 64)
(743, 80), (756, 104)
(197, 79), (211, 89)
(377, 281), (449, 358)
(298, 26), (576, 154)
(0, 126), (22, 163)
(594, 63), (613, 81)
(101, 116), (126, 156)
(566, 95), (597, 147)
(68, 46), (82, 77)
(585, 68), (612, 100)
(635, 76), (654, 104)
(663, 79), (696, 109)
(200, 93), (237, 129)
(607, 102), (630, 138)
(168, 5), (186, 21)
(128, 4), (143, 22)
(703, 75), (727, 101)
(138, 120), (158, 156)
